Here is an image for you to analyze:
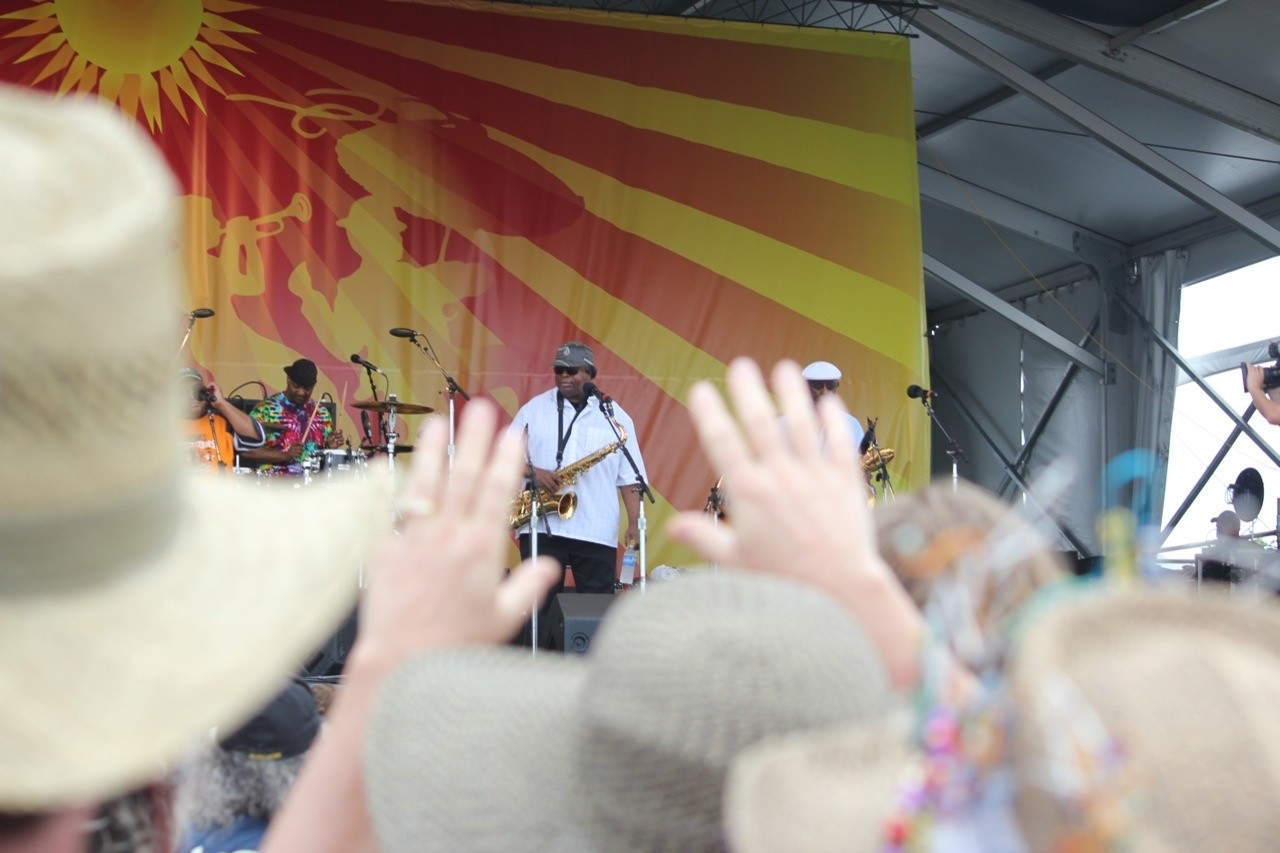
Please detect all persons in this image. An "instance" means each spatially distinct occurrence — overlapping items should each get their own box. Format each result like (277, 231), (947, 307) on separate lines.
(0, 81), (1280, 853)
(507, 340), (648, 594)
(240, 358), (344, 474)
(803, 361), (876, 501)
(174, 366), (267, 474)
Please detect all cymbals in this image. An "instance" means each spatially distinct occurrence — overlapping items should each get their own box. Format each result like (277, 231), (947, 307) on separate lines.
(350, 401), (435, 415)
(361, 442), (416, 456)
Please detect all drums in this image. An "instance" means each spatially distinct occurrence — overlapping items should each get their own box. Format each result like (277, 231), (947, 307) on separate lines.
(306, 449), (368, 483)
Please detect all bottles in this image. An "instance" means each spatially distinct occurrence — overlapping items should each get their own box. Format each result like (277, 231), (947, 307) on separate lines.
(620, 545), (636, 585)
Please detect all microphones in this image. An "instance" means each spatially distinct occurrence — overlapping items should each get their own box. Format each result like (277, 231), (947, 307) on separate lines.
(360, 410), (372, 444)
(859, 417), (878, 455)
(192, 386), (217, 402)
(390, 328), (423, 337)
(907, 384), (938, 399)
(583, 382), (613, 403)
(186, 308), (215, 317)
(350, 354), (384, 376)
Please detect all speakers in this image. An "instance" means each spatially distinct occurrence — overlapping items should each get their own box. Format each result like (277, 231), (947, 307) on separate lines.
(304, 613), (358, 675)
(538, 593), (617, 656)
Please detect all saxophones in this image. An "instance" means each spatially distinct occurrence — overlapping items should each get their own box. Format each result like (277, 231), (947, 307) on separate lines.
(506, 398), (628, 530)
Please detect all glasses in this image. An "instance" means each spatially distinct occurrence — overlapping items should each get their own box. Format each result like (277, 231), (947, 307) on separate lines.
(553, 368), (578, 375)
(813, 383), (835, 390)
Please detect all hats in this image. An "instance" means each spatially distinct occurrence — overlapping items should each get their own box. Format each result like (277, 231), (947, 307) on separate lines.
(802, 361), (842, 380)
(214, 678), (322, 762)
(283, 359), (317, 386)
(177, 367), (201, 385)
(722, 575), (1280, 853)
(549, 342), (594, 369)
(362, 570), (889, 853)
(0, 85), (400, 813)
